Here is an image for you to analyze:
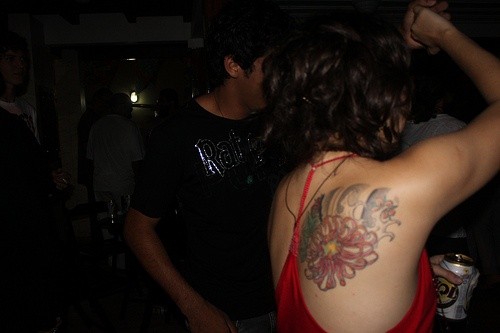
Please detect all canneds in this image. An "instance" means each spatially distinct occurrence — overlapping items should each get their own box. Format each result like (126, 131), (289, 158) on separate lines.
(433, 254), (480, 321)
(52, 169), (73, 193)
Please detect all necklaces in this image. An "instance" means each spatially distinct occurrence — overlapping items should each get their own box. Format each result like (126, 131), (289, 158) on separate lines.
(213, 89), (227, 120)
(283, 153), (350, 231)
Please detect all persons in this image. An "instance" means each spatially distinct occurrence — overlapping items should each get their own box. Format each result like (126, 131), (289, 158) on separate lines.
(121, 6), (290, 333)
(86, 92), (149, 239)
(1, 30), (74, 333)
(399, 66), (466, 145)
(262, 6), (499, 333)
(76, 87), (118, 239)
